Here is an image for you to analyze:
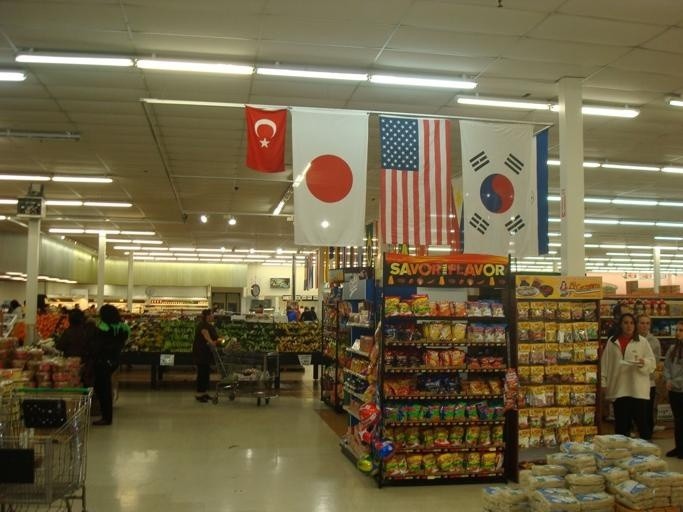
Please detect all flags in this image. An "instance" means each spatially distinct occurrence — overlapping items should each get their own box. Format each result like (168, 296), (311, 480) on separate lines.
(291, 107), (368, 248)
(457, 119), (539, 258)
(243, 105), (289, 173)
(459, 129), (550, 255)
(378, 115), (452, 248)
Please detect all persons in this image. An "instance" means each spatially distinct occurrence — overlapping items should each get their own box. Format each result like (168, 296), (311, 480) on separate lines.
(600, 313), (657, 441)
(636, 313), (662, 437)
(606, 302), (625, 339)
(287, 302), (318, 321)
(192, 309), (221, 403)
(1, 294), (131, 426)
(664, 319), (683, 461)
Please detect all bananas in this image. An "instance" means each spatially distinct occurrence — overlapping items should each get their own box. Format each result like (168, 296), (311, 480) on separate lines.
(160, 320), (196, 352)
(217, 320), (322, 352)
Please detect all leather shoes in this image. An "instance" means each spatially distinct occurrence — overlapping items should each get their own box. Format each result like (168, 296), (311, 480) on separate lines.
(93, 419), (111, 425)
(667, 448), (678, 457)
(196, 394), (213, 403)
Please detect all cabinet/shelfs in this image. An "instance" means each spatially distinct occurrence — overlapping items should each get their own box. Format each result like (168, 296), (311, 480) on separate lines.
(145, 297), (208, 310)
(322, 268), (683, 491)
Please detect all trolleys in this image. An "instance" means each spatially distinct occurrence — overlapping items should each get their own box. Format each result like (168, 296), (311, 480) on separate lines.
(208, 344), (282, 407)
(0, 385), (95, 512)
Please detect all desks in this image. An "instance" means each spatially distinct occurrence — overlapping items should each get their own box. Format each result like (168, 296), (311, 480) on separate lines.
(117, 352), (322, 390)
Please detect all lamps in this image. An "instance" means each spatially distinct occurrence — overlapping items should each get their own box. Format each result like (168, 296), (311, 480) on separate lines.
(0, 48), (683, 274)
